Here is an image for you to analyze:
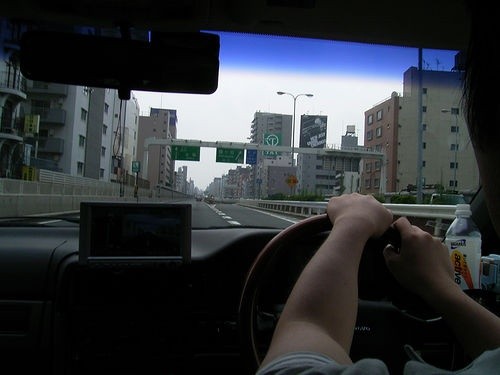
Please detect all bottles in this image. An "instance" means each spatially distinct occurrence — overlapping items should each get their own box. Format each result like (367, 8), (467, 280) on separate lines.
(445, 204), (482, 290)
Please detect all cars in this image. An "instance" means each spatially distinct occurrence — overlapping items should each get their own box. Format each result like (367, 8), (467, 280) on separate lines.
(196, 196), (202, 201)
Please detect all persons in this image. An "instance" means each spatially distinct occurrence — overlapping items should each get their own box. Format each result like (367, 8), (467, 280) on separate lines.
(254, 0), (500, 375)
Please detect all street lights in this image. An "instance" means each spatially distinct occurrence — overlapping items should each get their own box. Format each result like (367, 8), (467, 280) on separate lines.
(441, 108), (458, 192)
(277, 91), (314, 166)
